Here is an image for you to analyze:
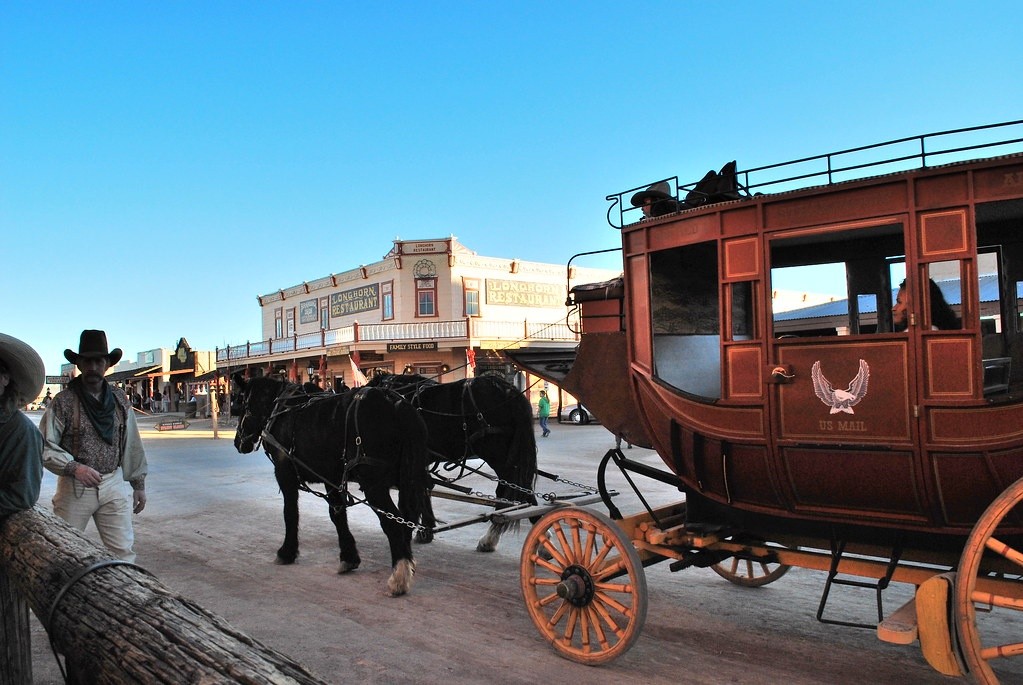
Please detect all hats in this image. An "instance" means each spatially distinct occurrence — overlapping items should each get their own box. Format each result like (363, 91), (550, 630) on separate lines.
(631, 182), (673, 206)
(47, 392), (51, 395)
(0, 333), (45, 408)
(64, 330), (122, 367)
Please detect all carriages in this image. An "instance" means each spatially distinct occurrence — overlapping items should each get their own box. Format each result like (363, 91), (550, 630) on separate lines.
(228, 119), (1023, 685)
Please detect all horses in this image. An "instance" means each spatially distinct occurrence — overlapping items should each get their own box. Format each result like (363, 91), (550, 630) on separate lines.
(227, 374), (437, 595)
(361, 370), (544, 552)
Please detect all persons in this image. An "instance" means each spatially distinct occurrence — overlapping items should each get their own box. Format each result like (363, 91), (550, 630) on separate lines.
(538, 391), (551, 437)
(892, 277), (962, 332)
(0, 333), (46, 524)
(43, 391), (53, 411)
(631, 182), (674, 221)
(38, 329), (147, 563)
(127, 388), (195, 413)
(218, 394), (224, 414)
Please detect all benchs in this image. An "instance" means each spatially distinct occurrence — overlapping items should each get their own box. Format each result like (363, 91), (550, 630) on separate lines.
(981, 333), (1023, 390)
(570, 275), (623, 333)
(652, 333), (752, 399)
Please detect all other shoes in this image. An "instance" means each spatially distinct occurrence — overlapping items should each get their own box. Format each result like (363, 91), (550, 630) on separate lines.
(541, 434), (545, 437)
(545, 430), (551, 437)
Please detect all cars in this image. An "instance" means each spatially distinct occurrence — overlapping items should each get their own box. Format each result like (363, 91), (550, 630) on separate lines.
(557, 403), (599, 425)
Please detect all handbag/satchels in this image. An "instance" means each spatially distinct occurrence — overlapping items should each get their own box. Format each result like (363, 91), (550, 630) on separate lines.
(686, 161), (738, 206)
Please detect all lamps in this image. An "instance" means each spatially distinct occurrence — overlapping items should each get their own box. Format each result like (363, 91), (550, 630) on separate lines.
(439, 363), (449, 374)
(405, 365), (414, 375)
